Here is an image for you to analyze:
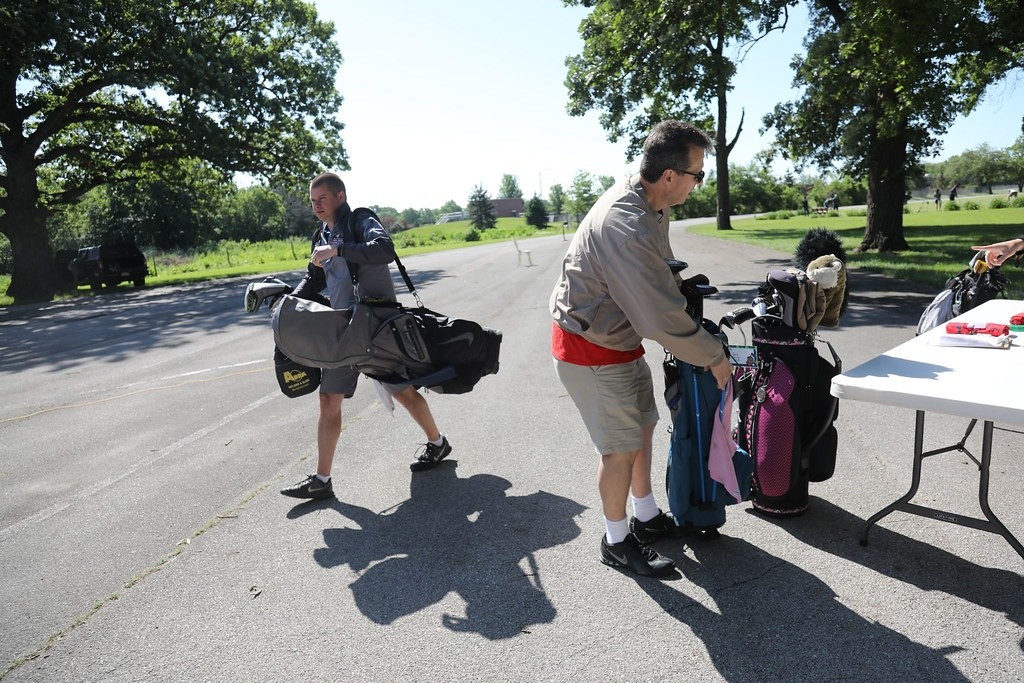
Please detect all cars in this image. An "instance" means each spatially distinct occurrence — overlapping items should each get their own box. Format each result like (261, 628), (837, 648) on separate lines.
(55, 239), (151, 292)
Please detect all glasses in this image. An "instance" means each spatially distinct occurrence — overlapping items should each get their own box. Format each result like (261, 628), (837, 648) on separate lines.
(677, 168), (705, 184)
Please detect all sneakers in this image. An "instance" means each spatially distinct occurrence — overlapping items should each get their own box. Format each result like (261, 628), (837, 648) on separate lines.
(280, 473), (335, 499)
(410, 435), (452, 472)
(629, 509), (695, 547)
(599, 532), (676, 578)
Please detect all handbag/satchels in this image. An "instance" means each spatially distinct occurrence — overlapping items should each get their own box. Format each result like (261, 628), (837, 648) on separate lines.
(271, 293), (504, 394)
(915, 267), (1012, 337)
(245, 274), (331, 398)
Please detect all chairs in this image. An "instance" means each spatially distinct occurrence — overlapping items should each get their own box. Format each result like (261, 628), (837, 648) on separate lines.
(513, 238), (534, 267)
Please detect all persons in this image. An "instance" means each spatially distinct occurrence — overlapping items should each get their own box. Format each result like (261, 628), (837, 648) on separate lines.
(745, 355), (754, 366)
(802, 193), (840, 217)
(1008, 189), (1018, 201)
(281, 173), (452, 500)
(933, 183), (958, 211)
(970, 235), (1024, 269)
(549, 120), (734, 579)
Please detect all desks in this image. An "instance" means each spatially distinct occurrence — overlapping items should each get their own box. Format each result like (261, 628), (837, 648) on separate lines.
(828, 298), (1024, 560)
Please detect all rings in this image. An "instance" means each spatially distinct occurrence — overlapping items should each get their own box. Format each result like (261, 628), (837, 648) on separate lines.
(313, 258), (316, 262)
(723, 376), (727, 381)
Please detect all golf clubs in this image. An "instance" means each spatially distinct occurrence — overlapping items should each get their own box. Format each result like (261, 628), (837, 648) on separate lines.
(662, 256), (736, 349)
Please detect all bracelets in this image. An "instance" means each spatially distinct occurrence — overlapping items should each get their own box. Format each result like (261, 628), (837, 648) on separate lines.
(337, 245), (341, 257)
